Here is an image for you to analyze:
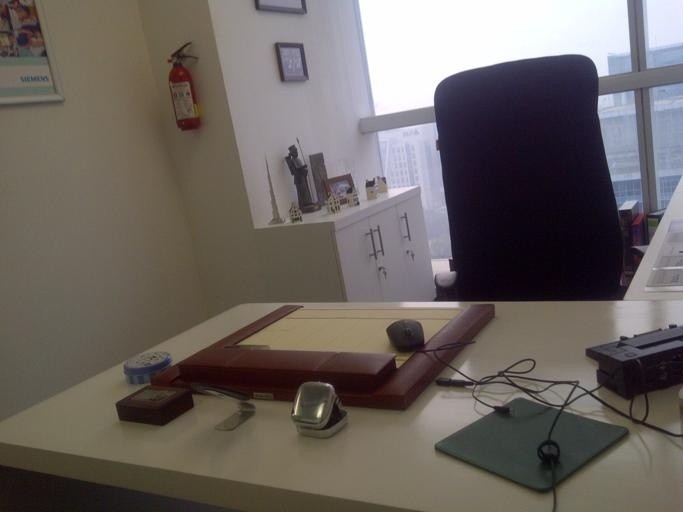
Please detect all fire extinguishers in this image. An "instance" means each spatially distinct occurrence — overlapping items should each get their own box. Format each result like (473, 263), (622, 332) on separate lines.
(166, 41), (200, 131)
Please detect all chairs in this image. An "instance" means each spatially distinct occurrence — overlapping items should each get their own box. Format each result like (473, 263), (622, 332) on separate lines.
(432, 55), (623, 300)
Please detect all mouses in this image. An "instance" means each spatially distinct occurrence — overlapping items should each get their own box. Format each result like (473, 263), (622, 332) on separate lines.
(385, 317), (424, 353)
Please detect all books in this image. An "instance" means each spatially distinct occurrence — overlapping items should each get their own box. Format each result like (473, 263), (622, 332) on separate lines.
(618, 199), (667, 272)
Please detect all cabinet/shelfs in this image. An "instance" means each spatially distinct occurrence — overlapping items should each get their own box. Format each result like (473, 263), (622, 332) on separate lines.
(256, 181), (437, 302)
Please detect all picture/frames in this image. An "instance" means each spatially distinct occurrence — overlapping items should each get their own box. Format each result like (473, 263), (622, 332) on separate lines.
(0, 1), (64, 108)
(275, 42), (309, 82)
(256, 0), (308, 15)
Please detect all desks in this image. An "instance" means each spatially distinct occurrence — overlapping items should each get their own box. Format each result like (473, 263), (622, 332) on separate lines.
(624, 176), (683, 301)
(2, 298), (682, 511)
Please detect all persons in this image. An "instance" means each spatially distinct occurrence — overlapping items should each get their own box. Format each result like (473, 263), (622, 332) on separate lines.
(287, 144), (312, 208)
